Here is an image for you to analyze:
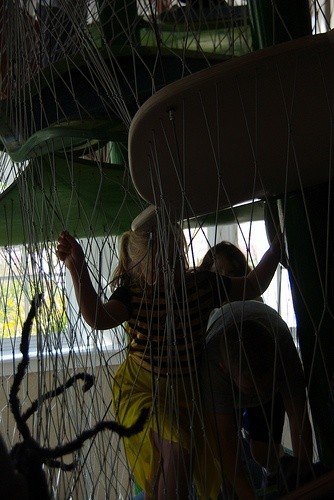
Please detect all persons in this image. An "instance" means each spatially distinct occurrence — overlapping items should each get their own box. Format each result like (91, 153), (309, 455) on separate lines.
(193, 241), (317, 500)
(56, 205), (285, 500)
